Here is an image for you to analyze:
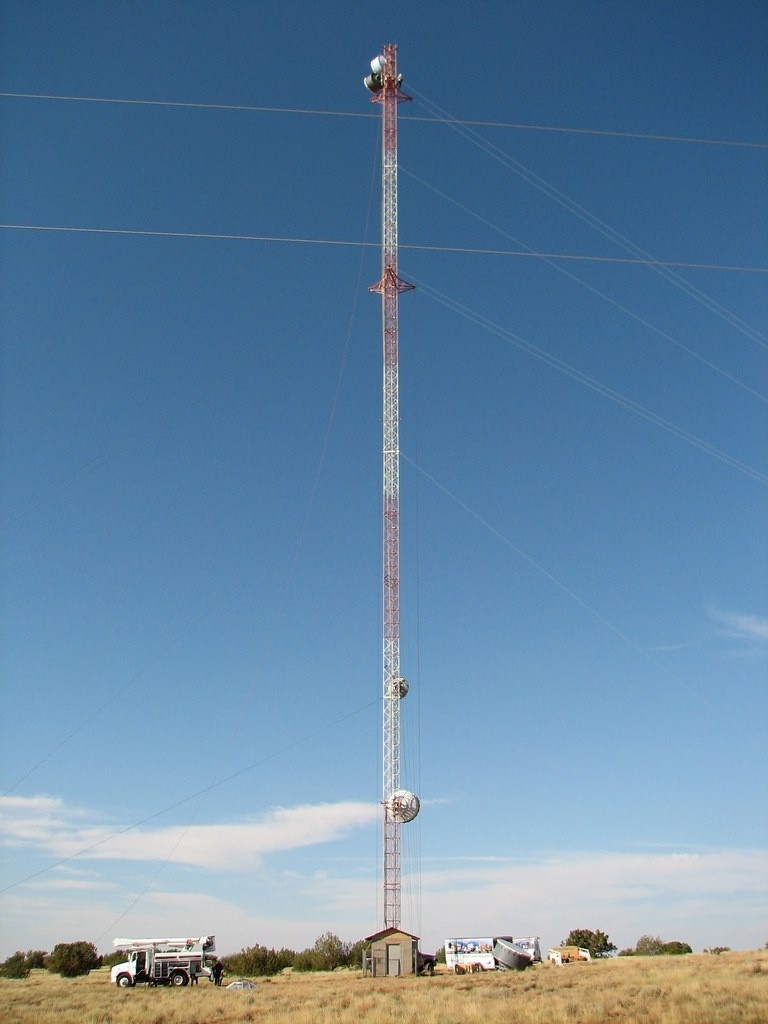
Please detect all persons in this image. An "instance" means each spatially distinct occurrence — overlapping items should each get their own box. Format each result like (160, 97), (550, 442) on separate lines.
(213, 961), (223, 986)
(424, 959), (438, 976)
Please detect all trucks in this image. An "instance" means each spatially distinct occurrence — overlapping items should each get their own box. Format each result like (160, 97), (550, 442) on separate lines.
(109, 944), (213, 986)
